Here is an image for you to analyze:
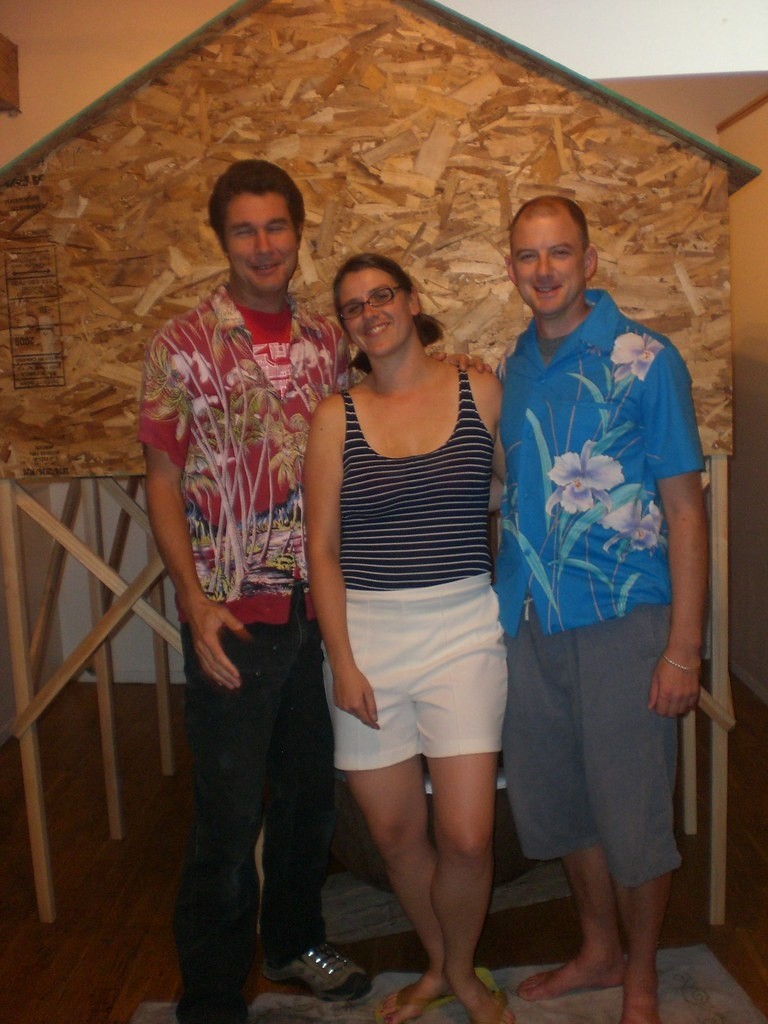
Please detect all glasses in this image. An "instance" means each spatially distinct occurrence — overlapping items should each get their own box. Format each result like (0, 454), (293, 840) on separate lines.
(338, 285), (405, 320)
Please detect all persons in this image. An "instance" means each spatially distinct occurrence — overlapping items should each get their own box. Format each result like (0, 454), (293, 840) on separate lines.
(496, 196), (706, 1024)
(137, 159), (492, 1024)
(305, 253), (515, 1024)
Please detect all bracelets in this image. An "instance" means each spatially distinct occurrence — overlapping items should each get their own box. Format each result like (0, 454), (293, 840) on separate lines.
(661, 655), (701, 670)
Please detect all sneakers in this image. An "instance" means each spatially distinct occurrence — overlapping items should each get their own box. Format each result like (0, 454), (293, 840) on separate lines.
(262, 942), (372, 1001)
